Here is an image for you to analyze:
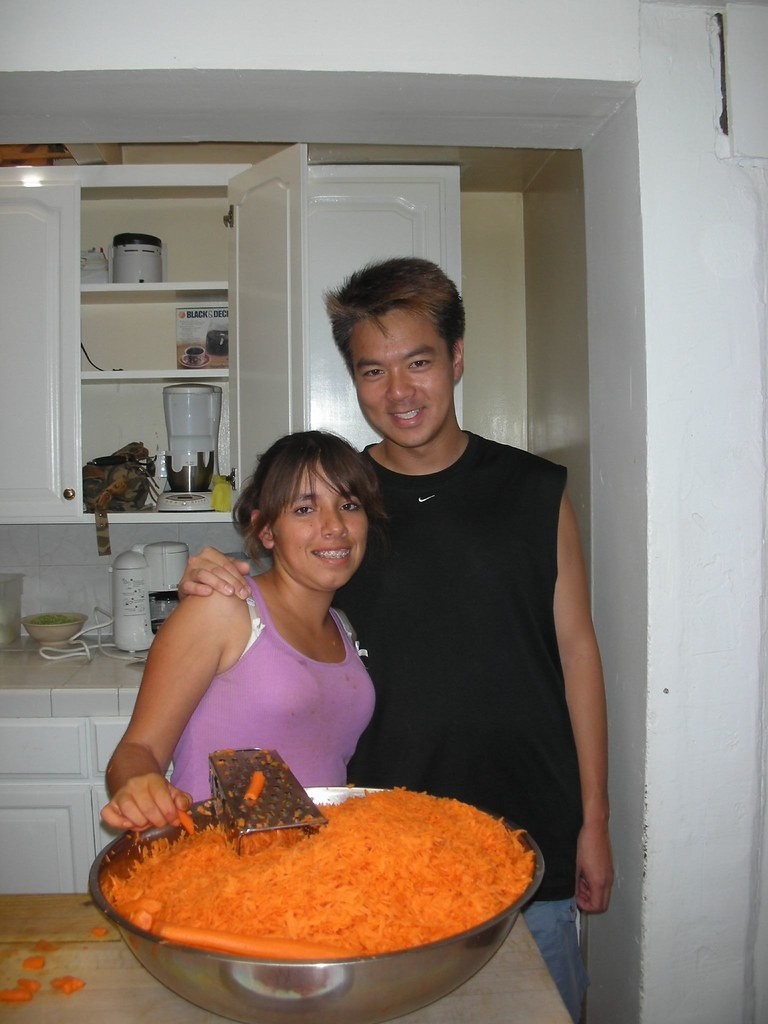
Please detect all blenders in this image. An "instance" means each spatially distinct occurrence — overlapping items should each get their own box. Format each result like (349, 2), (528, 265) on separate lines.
(143, 542), (190, 637)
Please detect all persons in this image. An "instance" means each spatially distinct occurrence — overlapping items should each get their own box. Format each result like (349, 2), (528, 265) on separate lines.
(100, 428), (379, 833)
(177, 256), (614, 1024)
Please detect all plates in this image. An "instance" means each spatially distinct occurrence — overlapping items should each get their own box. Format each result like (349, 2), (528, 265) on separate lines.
(178, 355), (211, 369)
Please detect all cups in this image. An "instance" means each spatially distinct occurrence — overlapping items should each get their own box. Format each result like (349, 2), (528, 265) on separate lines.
(182, 346), (206, 365)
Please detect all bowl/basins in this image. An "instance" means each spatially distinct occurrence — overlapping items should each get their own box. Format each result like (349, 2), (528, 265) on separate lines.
(88, 785), (545, 1024)
(21, 612), (88, 645)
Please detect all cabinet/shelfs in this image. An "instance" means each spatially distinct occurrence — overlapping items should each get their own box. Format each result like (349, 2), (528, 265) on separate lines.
(0, 720), (133, 894)
(0, 139), (464, 522)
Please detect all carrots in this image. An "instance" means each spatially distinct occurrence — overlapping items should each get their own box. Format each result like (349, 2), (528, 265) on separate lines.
(0, 927), (107, 1001)
(98, 767), (537, 960)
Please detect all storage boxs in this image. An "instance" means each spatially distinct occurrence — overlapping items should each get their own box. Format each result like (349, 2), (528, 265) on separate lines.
(174, 308), (229, 370)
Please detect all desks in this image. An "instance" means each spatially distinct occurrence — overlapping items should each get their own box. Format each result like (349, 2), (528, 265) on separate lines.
(0, 877), (572, 1024)
